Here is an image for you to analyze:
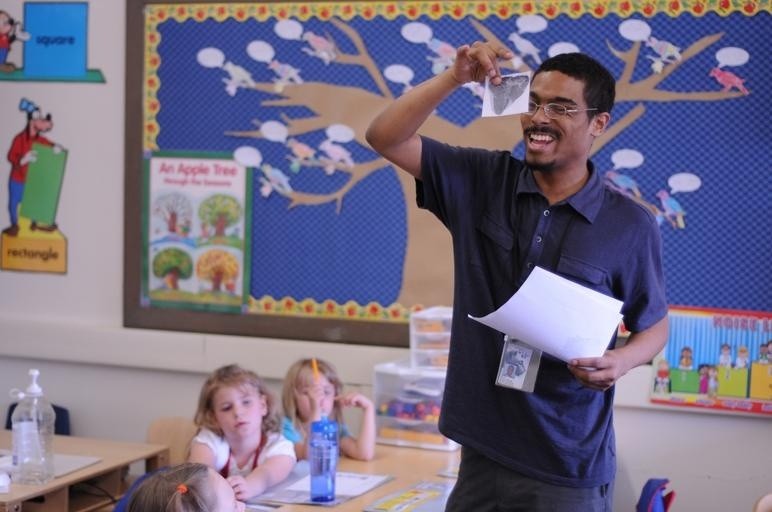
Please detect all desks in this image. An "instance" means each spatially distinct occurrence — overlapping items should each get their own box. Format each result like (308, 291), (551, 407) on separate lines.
(1, 426), (171, 510)
(266, 432), (462, 511)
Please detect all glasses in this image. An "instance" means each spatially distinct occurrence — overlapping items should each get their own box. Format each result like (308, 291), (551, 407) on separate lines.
(521, 100), (598, 120)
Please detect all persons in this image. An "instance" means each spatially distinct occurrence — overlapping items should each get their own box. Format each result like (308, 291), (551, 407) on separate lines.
(124, 462), (248, 511)
(366, 40), (670, 512)
(185, 364), (298, 501)
(280, 357), (378, 462)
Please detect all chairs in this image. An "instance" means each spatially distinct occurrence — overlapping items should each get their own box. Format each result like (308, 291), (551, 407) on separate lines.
(148, 416), (201, 467)
(7, 403), (70, 436)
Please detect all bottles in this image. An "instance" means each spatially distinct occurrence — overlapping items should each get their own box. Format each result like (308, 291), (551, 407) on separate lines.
(309, 416), (339, 503)
